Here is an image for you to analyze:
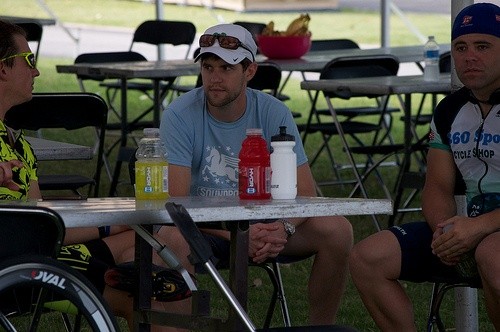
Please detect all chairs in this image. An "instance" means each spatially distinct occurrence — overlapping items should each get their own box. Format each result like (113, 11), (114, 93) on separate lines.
(0, 19), (483, 332)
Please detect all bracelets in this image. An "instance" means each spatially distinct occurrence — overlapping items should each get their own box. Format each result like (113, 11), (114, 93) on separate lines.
(98, 225), (110, 238)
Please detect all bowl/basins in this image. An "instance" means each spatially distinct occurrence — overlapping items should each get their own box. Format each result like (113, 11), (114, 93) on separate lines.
(255, 31), (311, 60)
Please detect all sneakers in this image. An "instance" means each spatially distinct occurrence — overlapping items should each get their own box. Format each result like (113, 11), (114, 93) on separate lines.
(105, 260), (198, 302)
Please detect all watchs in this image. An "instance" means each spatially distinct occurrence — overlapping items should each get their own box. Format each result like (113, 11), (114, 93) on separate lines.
(280, 219), (296, 238)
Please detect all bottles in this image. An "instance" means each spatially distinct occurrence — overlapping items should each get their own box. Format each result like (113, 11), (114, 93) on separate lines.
(238, 129), (272, 200)
(270, 126), (297, 200)
(423, 36), (440, 81)
(134, 128), (169, 200)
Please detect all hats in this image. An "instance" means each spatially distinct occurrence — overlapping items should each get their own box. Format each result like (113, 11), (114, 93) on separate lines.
(451, 2), (500, 40)
(193, 24), (257, 65)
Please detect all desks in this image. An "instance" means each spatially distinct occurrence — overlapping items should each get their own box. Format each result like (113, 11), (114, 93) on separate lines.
(25, 136), (94, 200)
(1, 196), (394, 332)
(301, 73), (451, 236)
(56, 43), (451, 192)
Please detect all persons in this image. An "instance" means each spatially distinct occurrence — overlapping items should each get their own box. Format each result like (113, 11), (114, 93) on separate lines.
(350, 6), (500, 332)
(0, 20), (158, 332)
(151, 23), (355, 332)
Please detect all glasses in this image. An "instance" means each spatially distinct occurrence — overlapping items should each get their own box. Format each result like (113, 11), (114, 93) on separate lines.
(0, 53), (35, 69)
(198, 33), (255, 62)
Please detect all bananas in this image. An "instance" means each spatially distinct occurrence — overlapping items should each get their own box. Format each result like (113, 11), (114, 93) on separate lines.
(263, 14), (311, 36)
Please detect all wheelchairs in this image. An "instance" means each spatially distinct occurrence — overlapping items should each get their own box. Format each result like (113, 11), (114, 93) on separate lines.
(0, 202), (122, 332)
